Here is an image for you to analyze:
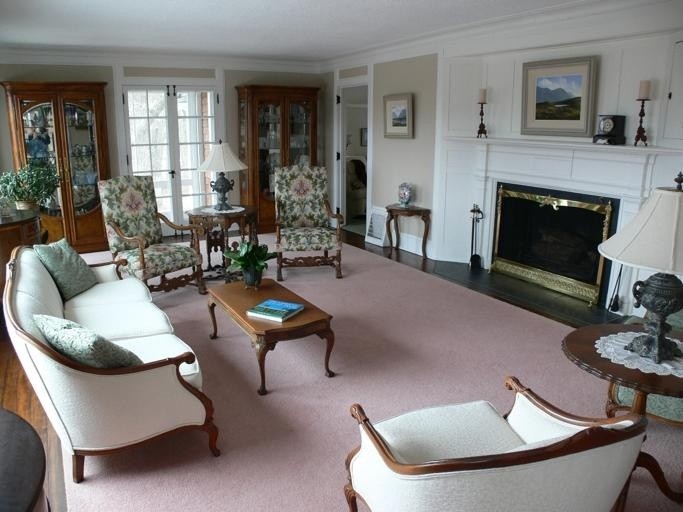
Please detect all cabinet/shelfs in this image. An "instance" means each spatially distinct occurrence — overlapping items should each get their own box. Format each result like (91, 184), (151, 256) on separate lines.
(0, 207), (41, 294)
(0, 79), (116, 255)
(232, 80), (322, 234)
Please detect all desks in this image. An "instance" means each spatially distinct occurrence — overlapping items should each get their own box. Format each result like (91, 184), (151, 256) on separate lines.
(0, 404), (55, 511)
(384, 202), (430, 260)
(559, 318), (682, 510)
(181, 202), (261, 286)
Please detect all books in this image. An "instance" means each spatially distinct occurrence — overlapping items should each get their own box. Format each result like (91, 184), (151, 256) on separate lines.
(246, 297), (305, 322)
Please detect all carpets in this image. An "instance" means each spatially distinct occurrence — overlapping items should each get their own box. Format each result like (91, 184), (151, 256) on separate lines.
(42, 222), (682, 512)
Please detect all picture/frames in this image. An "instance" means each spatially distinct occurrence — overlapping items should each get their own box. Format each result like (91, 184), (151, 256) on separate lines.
(518, 53), (603, 140)
(358, 127), (367, 148)
(380, 92), (416, 139)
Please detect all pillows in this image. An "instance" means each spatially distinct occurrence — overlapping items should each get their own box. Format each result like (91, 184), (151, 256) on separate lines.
(29, 308), (145, 370)
(30, 231), (102, 301)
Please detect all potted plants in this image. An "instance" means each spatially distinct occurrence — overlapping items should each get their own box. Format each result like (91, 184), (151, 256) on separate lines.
(219, 238), (279, 289)
(0, 164), (60, 211)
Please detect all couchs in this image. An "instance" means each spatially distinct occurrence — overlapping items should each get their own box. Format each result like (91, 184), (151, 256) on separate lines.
(1, 240), (221, 485)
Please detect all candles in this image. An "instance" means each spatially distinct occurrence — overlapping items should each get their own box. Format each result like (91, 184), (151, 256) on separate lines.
(640, 79), (650, 100)
(478, 87), (487, 105)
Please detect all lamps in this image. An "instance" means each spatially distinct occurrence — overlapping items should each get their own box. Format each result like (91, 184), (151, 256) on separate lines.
(344, 134), (353, 151)
(194, 137), (250, 211)
(592, 171), (682, 365)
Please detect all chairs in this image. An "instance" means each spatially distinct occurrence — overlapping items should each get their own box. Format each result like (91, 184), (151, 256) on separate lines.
(271, 159), (349, 289)
(333, 370), (649, 511)
(344, 156), (368, 225)
(95, 170), (211, 298)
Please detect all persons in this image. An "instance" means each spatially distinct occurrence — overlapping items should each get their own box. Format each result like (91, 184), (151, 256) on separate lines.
(27, 126), (50, 157)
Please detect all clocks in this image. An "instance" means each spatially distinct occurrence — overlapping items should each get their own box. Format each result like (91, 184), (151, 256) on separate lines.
(589, 111), (627, 147)
